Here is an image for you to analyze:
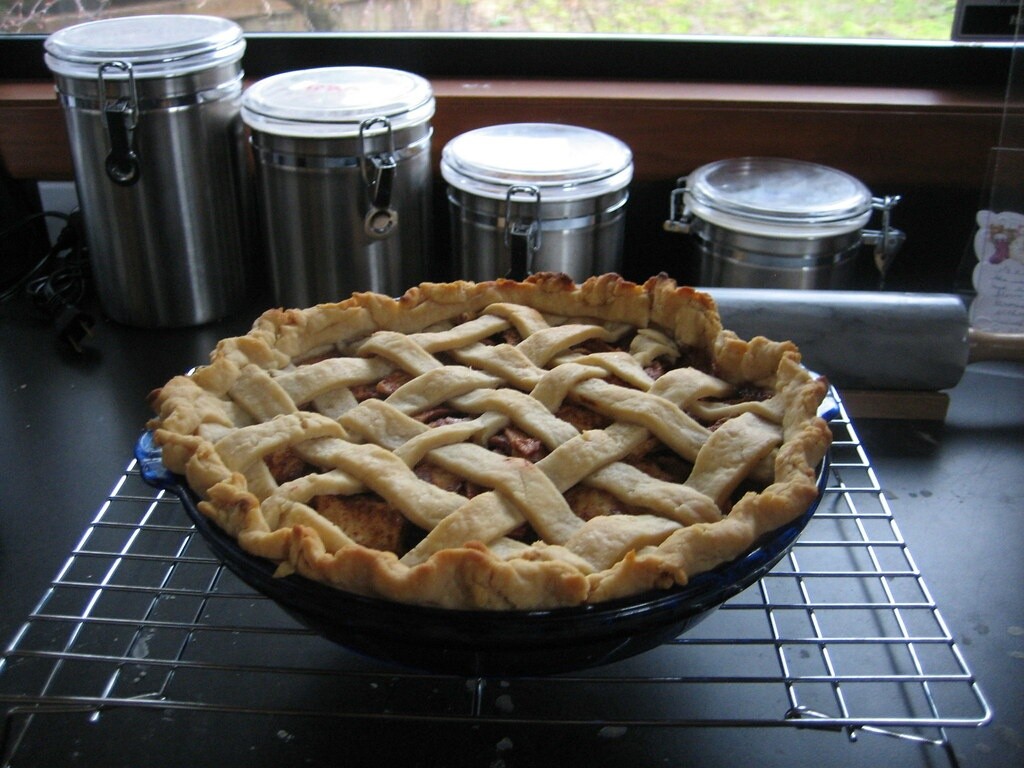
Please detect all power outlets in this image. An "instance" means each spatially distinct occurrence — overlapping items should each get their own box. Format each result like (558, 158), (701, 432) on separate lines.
(37, 182), (94, 260)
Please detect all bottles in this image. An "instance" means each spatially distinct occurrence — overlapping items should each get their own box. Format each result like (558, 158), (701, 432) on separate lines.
(440, 123), (634, 284)
(43, 14), (246, 329)
(664, 156), (905, 291)
(239, 66), (436, 312)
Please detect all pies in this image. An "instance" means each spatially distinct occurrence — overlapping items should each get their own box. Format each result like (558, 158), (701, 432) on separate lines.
(144, 273), (833, 613)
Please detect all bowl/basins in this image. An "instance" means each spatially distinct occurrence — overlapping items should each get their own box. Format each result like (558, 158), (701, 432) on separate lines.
(136, 395), (837, 675)
